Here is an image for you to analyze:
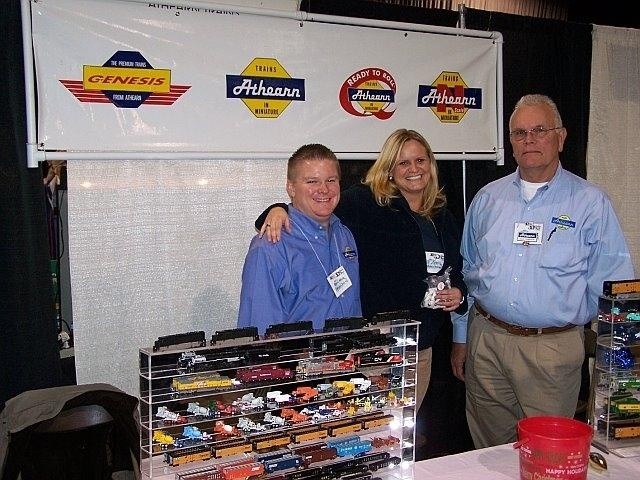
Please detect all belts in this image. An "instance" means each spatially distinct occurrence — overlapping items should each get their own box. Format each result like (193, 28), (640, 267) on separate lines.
(473, 301), (578, 337)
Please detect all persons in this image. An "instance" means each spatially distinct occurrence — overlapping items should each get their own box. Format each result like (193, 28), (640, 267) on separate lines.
(252, 128), (469, 459)
(448, 94), (638, 450)
(236, 142), (363, 338)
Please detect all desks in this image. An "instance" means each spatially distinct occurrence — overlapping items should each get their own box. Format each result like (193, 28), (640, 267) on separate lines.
(410, 439), (640, 480)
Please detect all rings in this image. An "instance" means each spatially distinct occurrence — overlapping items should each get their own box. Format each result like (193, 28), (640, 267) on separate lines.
(265, 224), (271, 227)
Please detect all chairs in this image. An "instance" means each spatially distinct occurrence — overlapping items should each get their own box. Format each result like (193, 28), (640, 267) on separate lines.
(2, 389), (140, 480)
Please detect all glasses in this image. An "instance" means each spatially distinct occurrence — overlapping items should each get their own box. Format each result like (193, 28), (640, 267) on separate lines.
(510, 125), (562, 141)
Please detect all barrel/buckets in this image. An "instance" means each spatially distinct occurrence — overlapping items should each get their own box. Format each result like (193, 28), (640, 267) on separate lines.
(513, 415), (595, 480)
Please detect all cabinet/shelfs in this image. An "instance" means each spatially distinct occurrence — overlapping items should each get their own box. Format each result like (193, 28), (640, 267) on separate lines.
(139, 320), (422, 480)
(593, 298), (640, 458)
